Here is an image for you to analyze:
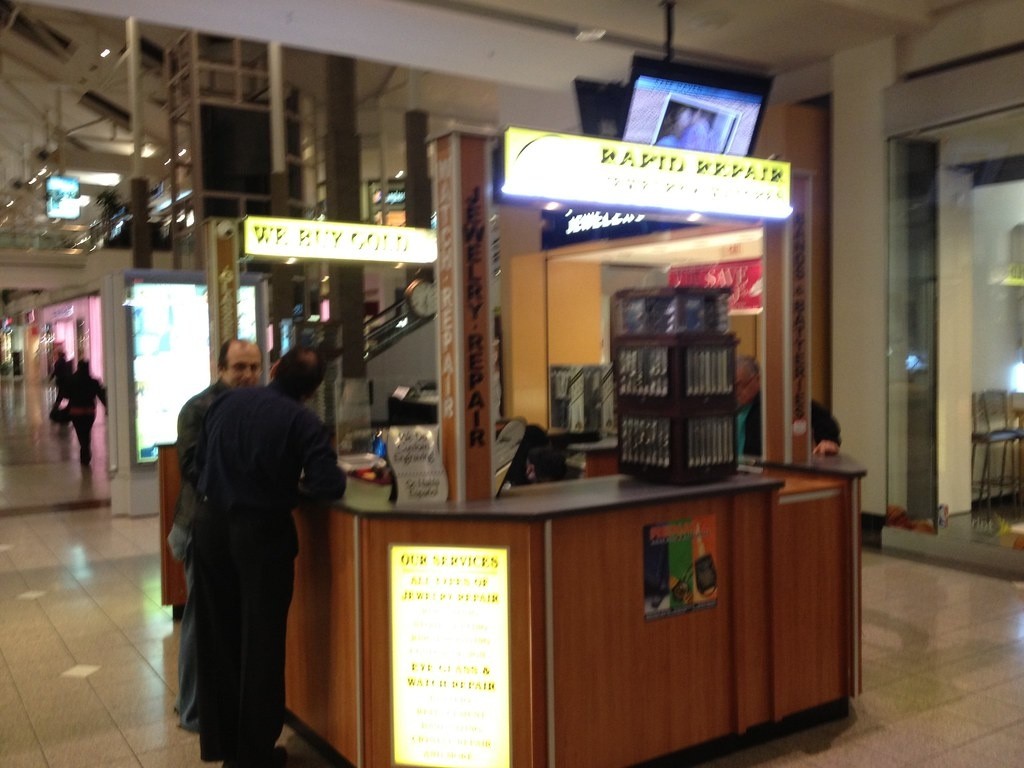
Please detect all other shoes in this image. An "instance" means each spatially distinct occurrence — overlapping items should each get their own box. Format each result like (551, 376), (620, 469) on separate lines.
(271, 746), (287, 766)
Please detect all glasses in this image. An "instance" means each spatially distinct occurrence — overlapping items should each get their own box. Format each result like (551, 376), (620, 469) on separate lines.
(736, 371), (758, 392)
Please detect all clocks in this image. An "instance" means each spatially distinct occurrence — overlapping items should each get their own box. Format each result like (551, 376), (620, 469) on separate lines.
(405, 280), (439, 320)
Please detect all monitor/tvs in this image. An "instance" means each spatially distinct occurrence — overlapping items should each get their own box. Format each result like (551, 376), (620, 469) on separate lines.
(46, 177), (81, 220)
(574, 54), (775, 156)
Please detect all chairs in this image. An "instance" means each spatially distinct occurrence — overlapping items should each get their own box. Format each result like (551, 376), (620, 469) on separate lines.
(972, 390), (1021, 519)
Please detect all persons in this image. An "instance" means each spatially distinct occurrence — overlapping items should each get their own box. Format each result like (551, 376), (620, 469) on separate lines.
(48, 352), (74, 420)
(188, 346), (349, 767)
(165, 339), (265, 733)
(526, 437), (571, 486)
(737, 355), (842, 457)
(56, 359), (106, 471)
